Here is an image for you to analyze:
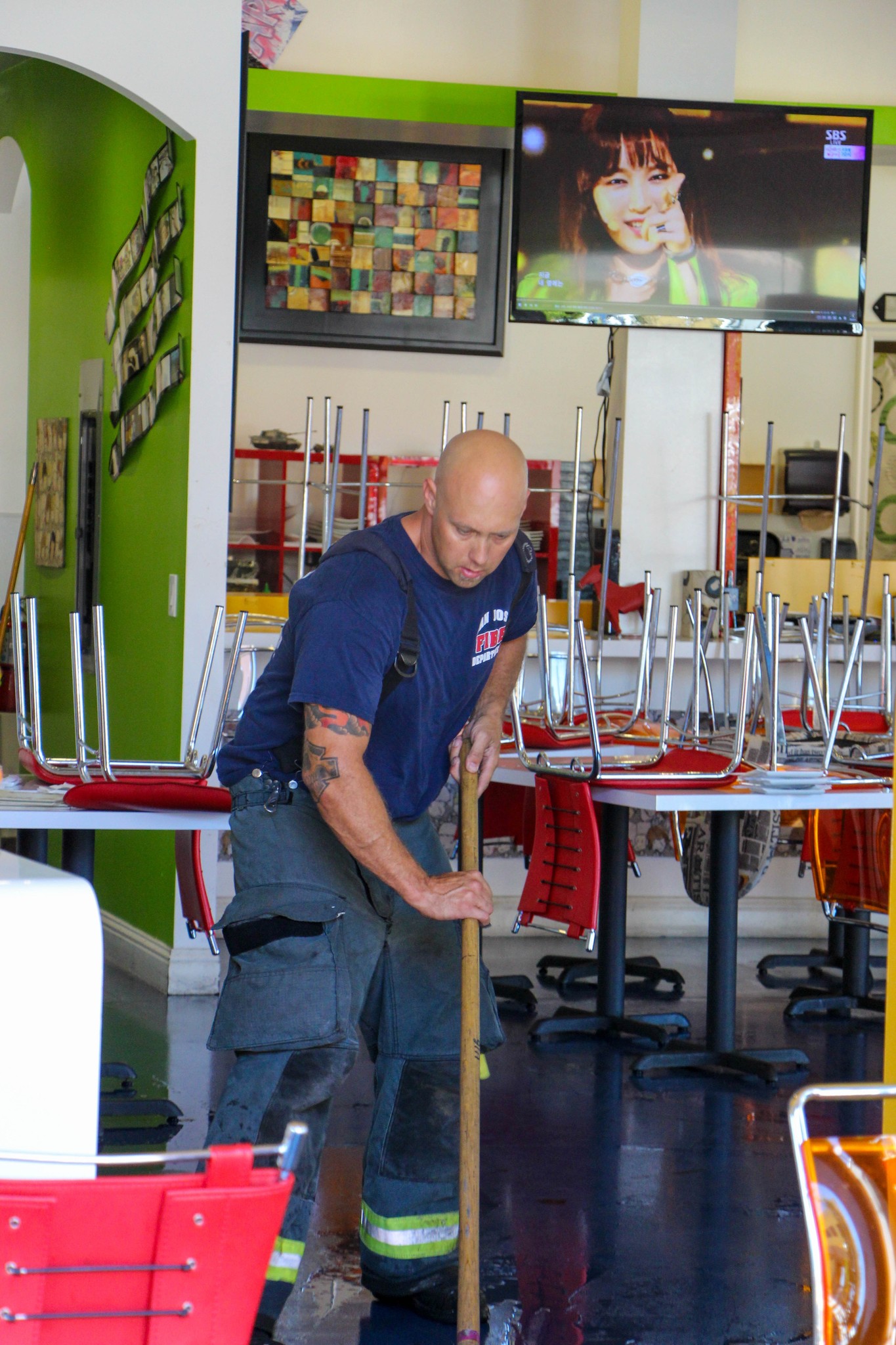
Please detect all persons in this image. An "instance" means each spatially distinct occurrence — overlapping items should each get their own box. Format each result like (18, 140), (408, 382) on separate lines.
(188, 430), (543, 1345)
(516, 105), (762, 307)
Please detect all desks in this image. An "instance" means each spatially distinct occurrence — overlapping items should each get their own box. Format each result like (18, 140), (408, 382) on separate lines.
(475, 753), (894, 1087)
(4, 776), (235, 1126)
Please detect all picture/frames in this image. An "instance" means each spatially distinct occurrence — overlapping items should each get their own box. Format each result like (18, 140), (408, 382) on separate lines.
(237, 128), (511, 357)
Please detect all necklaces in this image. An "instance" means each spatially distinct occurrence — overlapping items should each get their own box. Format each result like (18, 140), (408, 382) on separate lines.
(608, 254), (658, 287)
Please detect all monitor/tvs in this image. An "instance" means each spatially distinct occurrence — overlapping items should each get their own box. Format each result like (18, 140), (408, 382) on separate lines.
(510, 90), (874, 337)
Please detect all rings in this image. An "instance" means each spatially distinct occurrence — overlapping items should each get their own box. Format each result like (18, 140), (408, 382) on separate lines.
(656, 223), (667, 232)
(669, 195), (676, 205)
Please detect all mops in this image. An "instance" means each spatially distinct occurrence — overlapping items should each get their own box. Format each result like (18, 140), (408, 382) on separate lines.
(454, 736), (523, 1345)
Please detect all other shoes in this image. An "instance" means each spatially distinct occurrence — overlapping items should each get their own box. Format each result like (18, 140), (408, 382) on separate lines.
(371, 1274), (491, 1328)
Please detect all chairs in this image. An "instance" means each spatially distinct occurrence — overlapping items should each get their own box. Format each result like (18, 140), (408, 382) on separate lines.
(11, 395), (896, 959)
(0, 1123), (312, 1345)
